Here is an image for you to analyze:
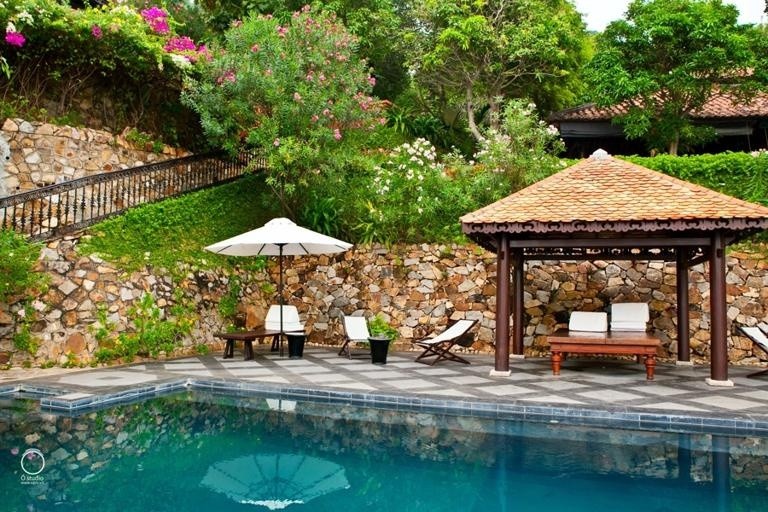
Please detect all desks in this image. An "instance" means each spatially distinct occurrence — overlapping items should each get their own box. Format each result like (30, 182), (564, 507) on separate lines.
(548, 327), (661, 382)
(213, 330), (281, 360)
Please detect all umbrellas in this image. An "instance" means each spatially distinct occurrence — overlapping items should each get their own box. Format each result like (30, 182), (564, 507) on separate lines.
(204, 217), (356, 357)
(197, 397), (351, 512)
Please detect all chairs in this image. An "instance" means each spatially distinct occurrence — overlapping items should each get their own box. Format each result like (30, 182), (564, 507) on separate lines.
(339, 314), (371, 364)
(264, 304), (305, 358)
(735, 320), (768, 378)
(411, 317), (479, 366)
